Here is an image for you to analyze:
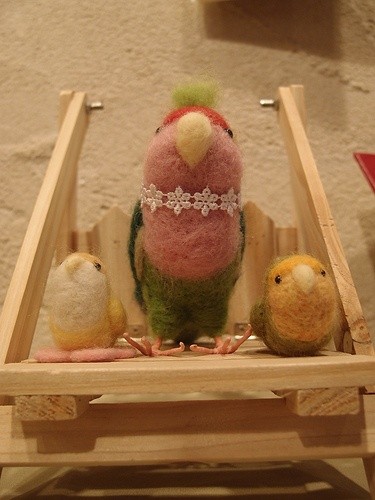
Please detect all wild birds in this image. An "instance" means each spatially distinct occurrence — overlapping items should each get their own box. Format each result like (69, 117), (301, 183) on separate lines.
(250, 254), (344, 357)
(47, 251), (129, 351)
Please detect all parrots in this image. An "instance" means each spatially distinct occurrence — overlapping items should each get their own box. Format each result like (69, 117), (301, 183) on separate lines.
(121, 81), (253, 357)
